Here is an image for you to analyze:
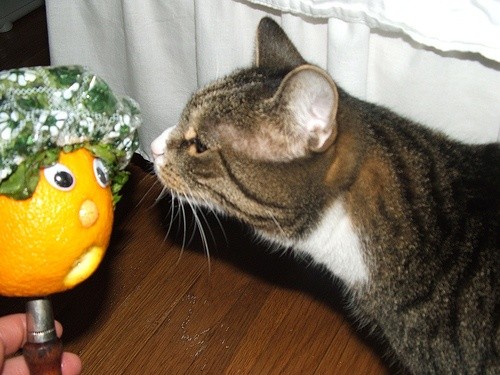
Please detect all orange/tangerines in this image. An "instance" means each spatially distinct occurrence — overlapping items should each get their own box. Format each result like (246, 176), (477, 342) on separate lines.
(0, 144), (115, 297)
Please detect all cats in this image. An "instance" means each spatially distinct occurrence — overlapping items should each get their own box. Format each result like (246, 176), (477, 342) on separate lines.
(136, 15), (500, 375)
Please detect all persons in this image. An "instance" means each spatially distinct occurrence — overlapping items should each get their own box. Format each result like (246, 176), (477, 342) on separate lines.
(0, 313), (82, 375)
